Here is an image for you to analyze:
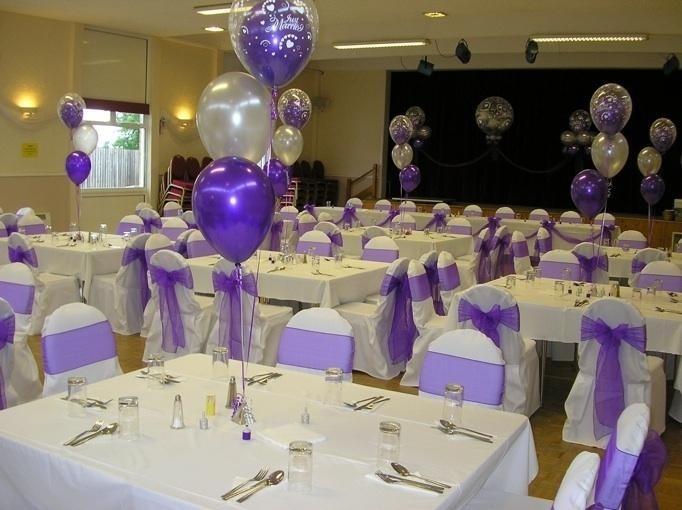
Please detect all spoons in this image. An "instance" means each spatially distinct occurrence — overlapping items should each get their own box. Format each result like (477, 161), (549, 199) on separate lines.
(388, 462), (451, 491)
(236, 469), (284, 504)
(653, 301), (681, 317)
(240, 372), (278, 381)
(75, 421), (117, 449)
(439, 419), (492, 439)
(339, 395), (383, 408)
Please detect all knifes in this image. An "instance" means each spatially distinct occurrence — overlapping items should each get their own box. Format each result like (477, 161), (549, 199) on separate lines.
(84, 401), (108, 409)
(353, 397), (384, 410)
(248, 373), (279, 386)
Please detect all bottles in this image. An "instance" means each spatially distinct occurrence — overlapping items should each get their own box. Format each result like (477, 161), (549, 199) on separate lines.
(300, 405), (311, 425)
(241, 423), (253, 443)
(205, 392), (216, 416)
(224, 375), (237, 409)
(170, 394), (184, 430)
(199, 411), (211, 432)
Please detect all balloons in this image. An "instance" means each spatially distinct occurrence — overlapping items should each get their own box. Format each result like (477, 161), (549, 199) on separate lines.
(57, 92), (87, 129)
(387, 105), (433, 193)
(475, 96), (517, 145)
(229, 0), (321, 88)
(195, 72), (312, 201)
(65, 151), (92, 186)
(240, 1), (313, 85)
(191, 156), (276, 264)
(73, 123), (98, 156)
(558, 83), (676, 225)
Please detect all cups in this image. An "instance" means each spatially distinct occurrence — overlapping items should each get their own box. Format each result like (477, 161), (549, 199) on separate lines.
(503, 265), (663, 309)
(378, 421), (401, 467)
(145, 353), (165, 391)
(15, 220), (140, 248)
(288, 440), (313, 494)
(66, 376), (88, 418)
(325, 366), (343, 407)
(117, 395), (141, 441)
(250, 238), (353, 274)
(344, 217), (456, 240)
(211, 348), (231, 381)
(445, 382), (464, 425)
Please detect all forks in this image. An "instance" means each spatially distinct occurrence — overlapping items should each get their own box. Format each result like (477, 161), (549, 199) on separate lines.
(372, 470), (443, 495)
(434, 425), (492, 445)
(219, 467), (268, 499)
(363, 398), (390, 409)
(87, 395), (113, 405)
(64, 418), (105, 445)
(257, 374), (283, 385)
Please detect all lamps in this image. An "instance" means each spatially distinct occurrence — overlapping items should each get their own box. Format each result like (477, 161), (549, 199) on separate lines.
(417, 57), (435, 76)
(434, 39), (471, 64)
(525, 39), (538, 64)
(330, 39), (431, 50)
(160, 109), (196, 142)
(528, 33), (648, 43)
(192, 0), (258, 15)
(10, 102), (45, 129)
